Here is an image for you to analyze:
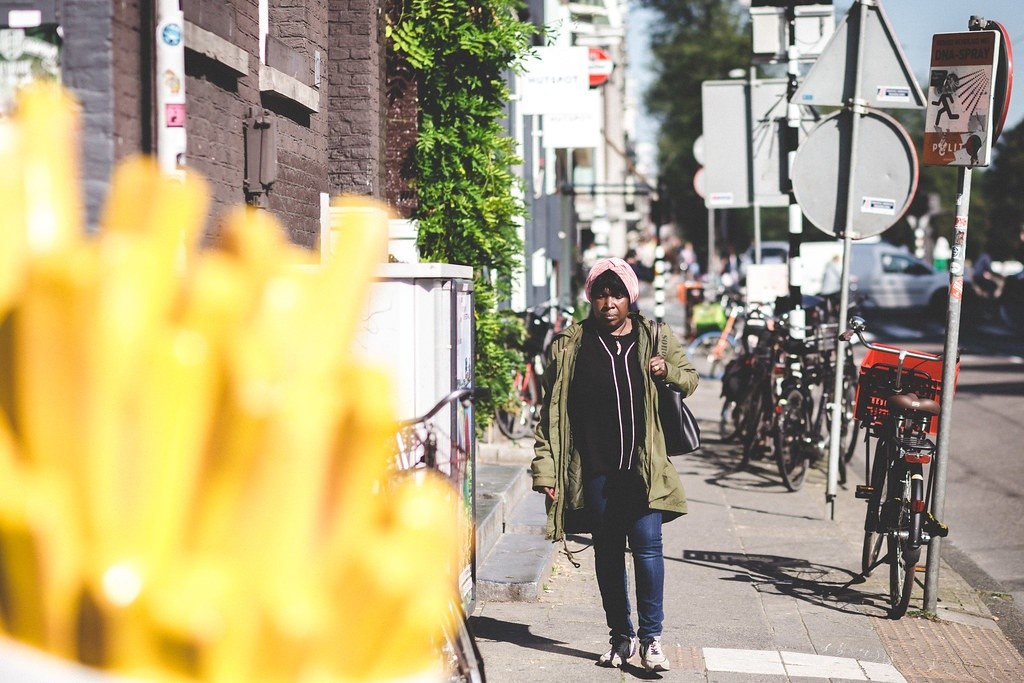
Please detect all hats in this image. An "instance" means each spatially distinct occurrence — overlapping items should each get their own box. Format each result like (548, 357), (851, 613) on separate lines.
(585, 258), (640, 304)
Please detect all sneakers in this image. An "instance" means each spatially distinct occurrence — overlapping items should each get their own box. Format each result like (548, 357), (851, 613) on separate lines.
(639, 635), (670, 673)
(599, 631), (636, 667)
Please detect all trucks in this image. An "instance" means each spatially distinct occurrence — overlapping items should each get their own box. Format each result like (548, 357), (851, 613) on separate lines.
(789, 236), (971, 334)
(736, 239), (792, 285)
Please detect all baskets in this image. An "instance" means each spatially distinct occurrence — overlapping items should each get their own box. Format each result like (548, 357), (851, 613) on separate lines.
(855, 375), (936, 433)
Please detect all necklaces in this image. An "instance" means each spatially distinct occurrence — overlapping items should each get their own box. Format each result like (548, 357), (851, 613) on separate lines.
(609, 321), (626, 354)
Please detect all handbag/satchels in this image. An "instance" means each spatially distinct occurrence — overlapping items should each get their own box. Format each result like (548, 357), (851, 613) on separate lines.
(719, 353), (747, 400)
(648, 319), (700, 455)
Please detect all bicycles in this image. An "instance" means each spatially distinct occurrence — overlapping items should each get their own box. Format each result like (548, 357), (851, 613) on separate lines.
(681, 287), (863, 493)
(382, 382), (494, 683)
(837, 316), (964, 618)
(491, 304), (577, 442)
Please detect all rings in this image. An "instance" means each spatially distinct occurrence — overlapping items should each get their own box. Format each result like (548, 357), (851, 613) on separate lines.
(654, 365), (659, 370)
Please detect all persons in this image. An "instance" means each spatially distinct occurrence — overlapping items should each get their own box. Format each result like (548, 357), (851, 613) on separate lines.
(531, 257), (701, 673)
(582, 242), (598, 277)
(821, 255), (856, 317)
